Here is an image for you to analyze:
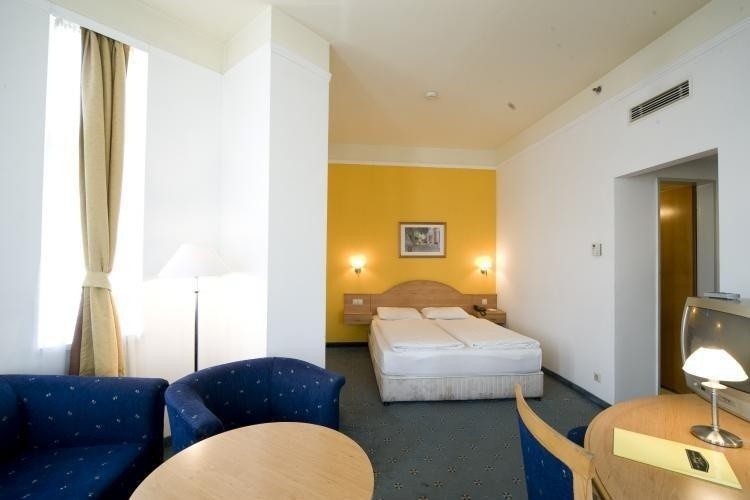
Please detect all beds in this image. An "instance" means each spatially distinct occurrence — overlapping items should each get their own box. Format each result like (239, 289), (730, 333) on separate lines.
(367, 279), (545, 407)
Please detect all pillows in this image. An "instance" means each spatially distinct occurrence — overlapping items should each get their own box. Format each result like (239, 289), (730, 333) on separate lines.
(421, 306), (469, 320)
(376, 306), (423, 321)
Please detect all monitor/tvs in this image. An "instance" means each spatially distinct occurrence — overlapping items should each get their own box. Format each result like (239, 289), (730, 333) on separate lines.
(680, 297), (750, 422)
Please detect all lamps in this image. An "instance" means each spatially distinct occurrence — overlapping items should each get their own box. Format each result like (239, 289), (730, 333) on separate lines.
(349, 254), (366, 277)
(681, 344), (749, 449)
(476, 255), (492, 274)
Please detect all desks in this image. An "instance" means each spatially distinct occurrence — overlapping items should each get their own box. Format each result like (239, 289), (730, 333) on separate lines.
(584, 392), (750, 500)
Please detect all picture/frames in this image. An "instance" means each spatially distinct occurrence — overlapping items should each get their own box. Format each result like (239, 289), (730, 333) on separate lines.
(398, 221), (447, 258)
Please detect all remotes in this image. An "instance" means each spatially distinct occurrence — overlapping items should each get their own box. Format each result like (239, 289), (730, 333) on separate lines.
(704, 292), (740, 299)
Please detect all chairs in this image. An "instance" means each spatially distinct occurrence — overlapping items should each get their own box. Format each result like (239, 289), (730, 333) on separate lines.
(163, 356), (345, 454)
(513, 381), (597, 500)
(0, 374), (170, 500)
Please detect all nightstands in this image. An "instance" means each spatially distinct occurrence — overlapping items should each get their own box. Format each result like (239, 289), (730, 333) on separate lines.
(343, 294), (371, 325)
(472, 293), (506, 324)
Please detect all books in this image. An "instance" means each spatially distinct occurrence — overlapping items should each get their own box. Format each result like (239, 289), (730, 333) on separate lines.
(614, 427), (742, 491)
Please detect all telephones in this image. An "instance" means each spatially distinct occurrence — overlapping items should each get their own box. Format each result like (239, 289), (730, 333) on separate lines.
(474, 305), (486, 311)
